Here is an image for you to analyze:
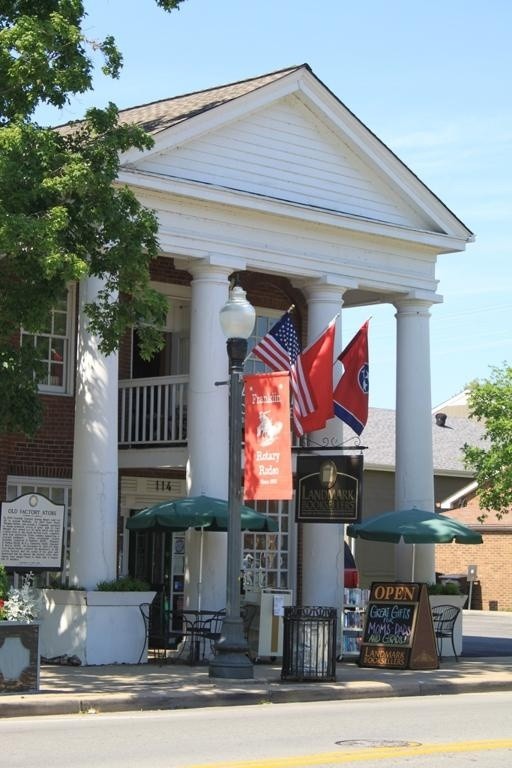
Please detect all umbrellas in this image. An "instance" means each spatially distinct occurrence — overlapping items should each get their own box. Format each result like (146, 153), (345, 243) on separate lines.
(346, 506), (483, 583)
(125, 494), (278, 640)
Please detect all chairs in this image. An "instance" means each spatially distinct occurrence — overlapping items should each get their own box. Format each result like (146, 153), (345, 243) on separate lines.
(138, 599), (258, 666)
(430, 604), (461, 664)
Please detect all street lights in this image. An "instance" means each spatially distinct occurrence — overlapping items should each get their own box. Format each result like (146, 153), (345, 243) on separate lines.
(208, 286), (256, 680)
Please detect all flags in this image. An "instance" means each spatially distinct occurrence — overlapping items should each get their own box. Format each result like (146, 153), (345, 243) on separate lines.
(292, 327), (335, 437)
(326, 322), (369, 436)
(253, 311), (318, 419)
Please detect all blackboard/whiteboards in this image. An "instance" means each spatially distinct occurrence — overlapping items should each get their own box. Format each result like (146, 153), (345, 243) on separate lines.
(361, 601), (419, 648)
